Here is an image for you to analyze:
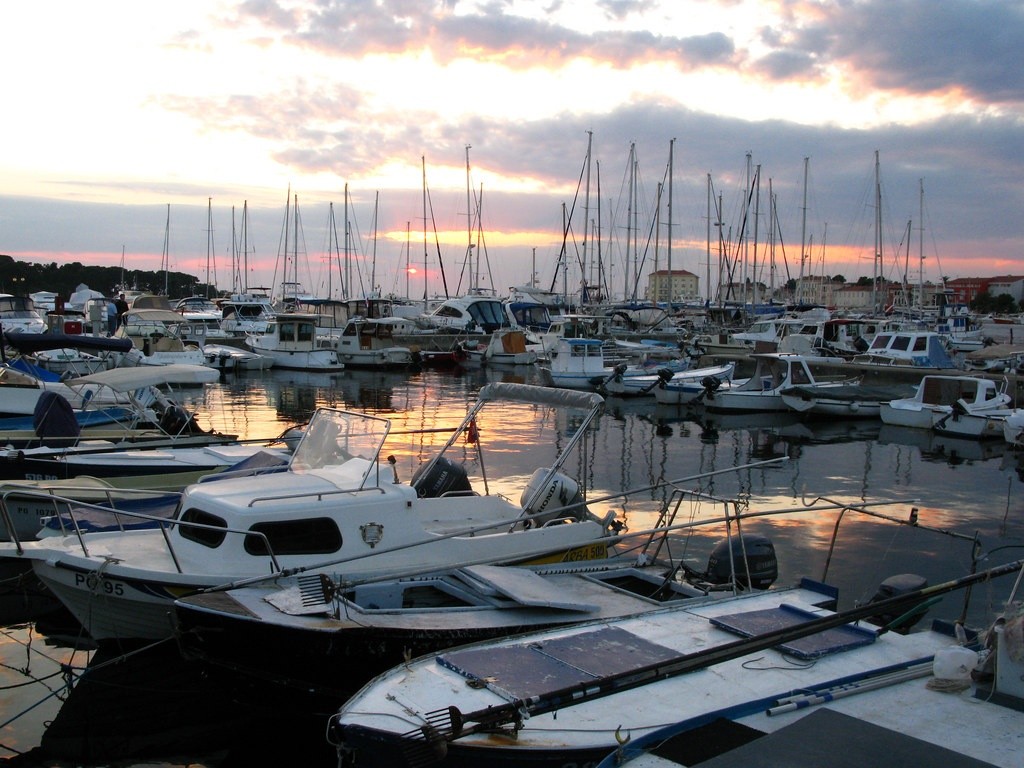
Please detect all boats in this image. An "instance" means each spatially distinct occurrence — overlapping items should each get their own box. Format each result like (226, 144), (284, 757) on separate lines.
(0, 293), (378, 589)
(596, 619), (1024, 768)
(778, 382), (920, 417)
(171, 490), (778, 698)
(325, 506), (1024, 768)
(0, 381), (627, 651)
(688, 353), (865, 413)
(317, 316), (419, 369)
(640, 368), (806, 404)
(878, 376), (1021, 436)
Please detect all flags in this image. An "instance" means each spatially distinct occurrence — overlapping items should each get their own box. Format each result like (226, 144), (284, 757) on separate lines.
(467, 420), (480, 443)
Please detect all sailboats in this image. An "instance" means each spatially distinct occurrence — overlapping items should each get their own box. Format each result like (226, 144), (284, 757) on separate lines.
(423, 131), (1023, 381)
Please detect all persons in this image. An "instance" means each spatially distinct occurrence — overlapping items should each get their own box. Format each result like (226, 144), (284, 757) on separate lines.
(107, 294), (129, 336)
(593, 318), (598, 334)
